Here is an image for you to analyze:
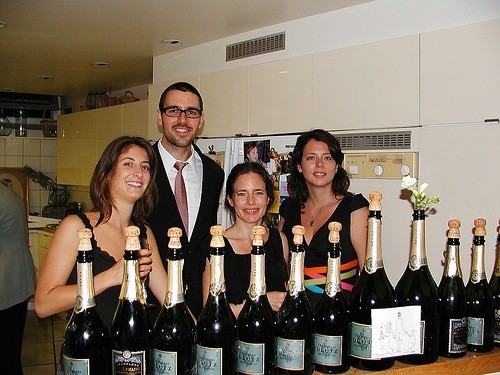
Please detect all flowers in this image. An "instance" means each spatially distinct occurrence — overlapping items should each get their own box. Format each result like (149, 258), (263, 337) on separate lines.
(401, 175), (441, 210)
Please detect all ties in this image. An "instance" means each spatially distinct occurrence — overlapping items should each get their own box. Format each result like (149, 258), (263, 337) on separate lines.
(173, 162), (188, 238)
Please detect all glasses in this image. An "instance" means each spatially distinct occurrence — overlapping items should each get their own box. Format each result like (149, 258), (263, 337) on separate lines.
(161, 105), (201, 118)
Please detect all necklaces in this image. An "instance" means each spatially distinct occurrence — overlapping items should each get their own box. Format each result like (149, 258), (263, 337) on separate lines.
(310, 203), (328, 226)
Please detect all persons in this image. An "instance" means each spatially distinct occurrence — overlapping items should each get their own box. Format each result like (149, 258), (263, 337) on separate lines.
(34, 135), (198, 327)
(0, 184), (36, 375)
(202, 161), (289, 321)
(246, 142), (262, 165)
(278, 129), (370, 308)
(149, 83), (224, 320)
(275, 152), (292, 173)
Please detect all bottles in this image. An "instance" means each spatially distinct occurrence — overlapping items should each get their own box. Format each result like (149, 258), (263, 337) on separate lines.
(489, 219), (500, 349)
(395, 209), (440, 365)
(153, 224), (197, 375)
(346, 193), (395, 372)
(235, 224), (276, 375)
(275, 225), (313, 374)
(194, 225), (237, 375)
(59, 227), (110, 375)
(0, 109), (12, 136)
(107, 227), (158, 375)
(15, 105), (28, 138)
(465, 217), (495, 355)
(437, 219), (468, 357)
(311, 222), (351, 374)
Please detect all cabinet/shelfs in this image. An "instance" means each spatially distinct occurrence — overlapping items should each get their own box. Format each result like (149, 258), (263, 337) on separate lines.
(29, 232), (69, 322)
(57, 100), (148, 186)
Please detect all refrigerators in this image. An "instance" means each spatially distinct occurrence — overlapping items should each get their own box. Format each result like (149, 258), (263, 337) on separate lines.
(194, 135), (304, 229)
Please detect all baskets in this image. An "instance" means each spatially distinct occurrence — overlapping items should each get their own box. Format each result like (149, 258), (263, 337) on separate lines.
(40, 105), (63, 138)
(119, 91), (140, 104)
(100, 87), (117, 107)
